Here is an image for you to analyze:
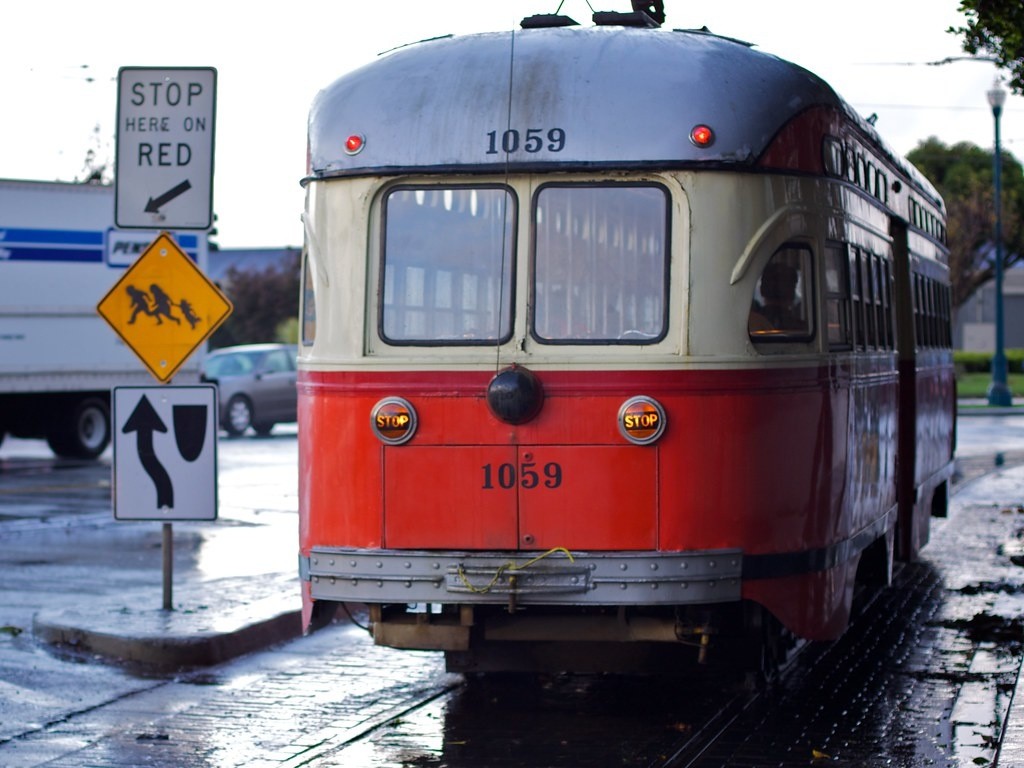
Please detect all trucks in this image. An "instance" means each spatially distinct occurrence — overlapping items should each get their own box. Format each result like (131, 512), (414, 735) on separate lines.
(0, 178), (213, 464)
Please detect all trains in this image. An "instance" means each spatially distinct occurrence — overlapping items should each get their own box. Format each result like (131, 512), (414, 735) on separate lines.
(298, 0), (960, 716)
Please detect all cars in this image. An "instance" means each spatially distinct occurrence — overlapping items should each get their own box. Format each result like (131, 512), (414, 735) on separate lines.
(207, 345), (299, 439)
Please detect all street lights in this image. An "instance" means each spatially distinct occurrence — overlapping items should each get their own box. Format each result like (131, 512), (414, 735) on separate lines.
(986, 77), (1012, 408)
